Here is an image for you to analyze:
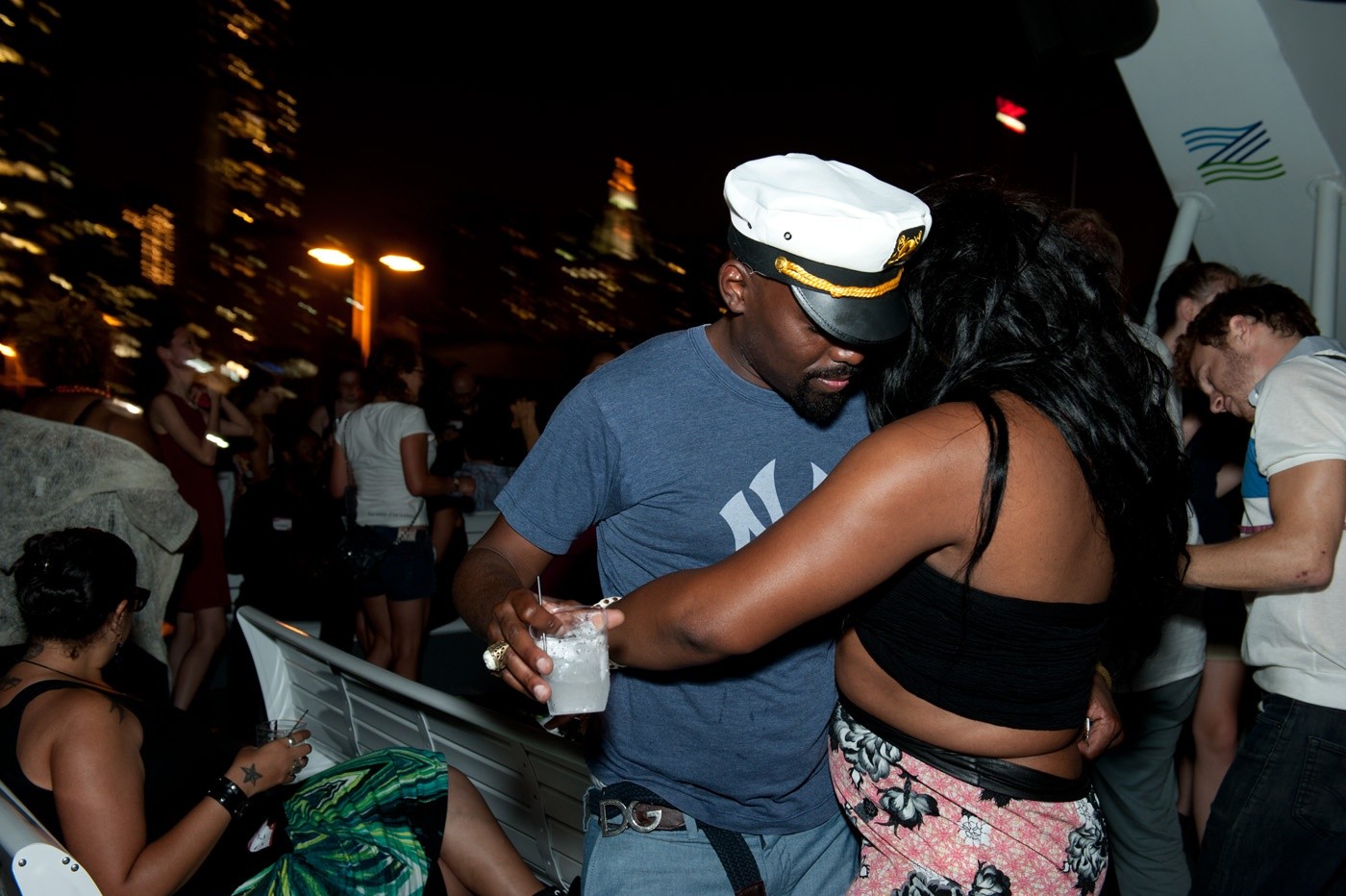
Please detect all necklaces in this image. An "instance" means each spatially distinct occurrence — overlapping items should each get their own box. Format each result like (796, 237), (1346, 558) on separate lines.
(166, 383), (199, 411)
(25, 655), (117, 690)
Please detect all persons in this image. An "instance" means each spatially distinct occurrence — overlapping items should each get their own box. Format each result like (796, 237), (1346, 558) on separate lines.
(0, 138), (1346, 896)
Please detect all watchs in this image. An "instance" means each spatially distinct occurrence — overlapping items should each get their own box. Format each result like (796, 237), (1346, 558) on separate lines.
(452, 476), (462, 491)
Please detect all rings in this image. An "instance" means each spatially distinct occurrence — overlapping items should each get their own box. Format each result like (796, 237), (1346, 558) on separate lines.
(285, 734), (296, 747)
(483, 641), (512, 672)
(292, 761), (303, 773)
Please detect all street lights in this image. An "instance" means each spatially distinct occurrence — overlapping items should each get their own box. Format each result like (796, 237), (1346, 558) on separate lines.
(306, 240), (426, 364)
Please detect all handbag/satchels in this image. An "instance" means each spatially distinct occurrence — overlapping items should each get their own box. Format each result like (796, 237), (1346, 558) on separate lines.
(338, 525), (400, 578)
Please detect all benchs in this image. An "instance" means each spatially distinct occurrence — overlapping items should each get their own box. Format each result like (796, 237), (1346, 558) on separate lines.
(237, 606), (597, 893)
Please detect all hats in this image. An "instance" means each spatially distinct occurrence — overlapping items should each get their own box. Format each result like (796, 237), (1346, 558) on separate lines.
(723, 153), (933, 347)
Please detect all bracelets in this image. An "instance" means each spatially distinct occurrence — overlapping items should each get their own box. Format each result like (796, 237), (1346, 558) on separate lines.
(205, 777), (249, 820)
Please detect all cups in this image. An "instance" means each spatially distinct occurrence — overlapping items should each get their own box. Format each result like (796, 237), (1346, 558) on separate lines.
(259, 718), (307, 762)
(528, 605), (610, 716)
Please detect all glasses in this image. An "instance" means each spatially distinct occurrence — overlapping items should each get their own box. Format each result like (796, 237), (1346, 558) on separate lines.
(125, 586), (150, 613)
(338, 381), (360, 388)
(451, 385), (476, 400)
